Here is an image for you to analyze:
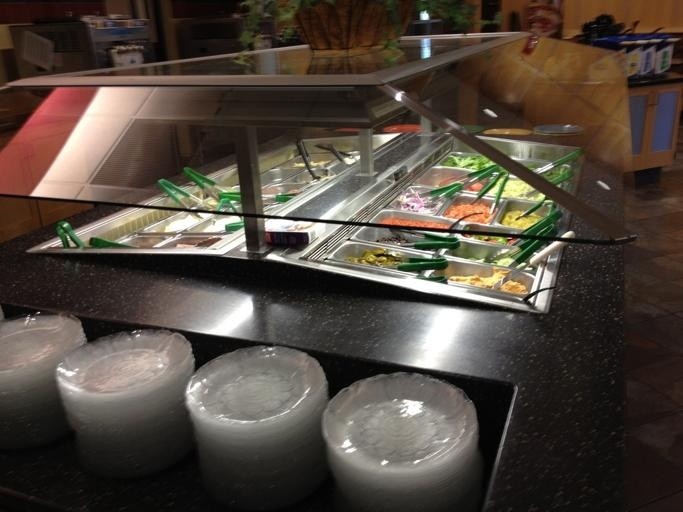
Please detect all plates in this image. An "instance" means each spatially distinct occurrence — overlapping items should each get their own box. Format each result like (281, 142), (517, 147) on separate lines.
(383, 123), (586, 136)
(321, 372), (480, 512)
(55, 327), (195, 476)
(186, 344), (329, 499)
(2, 313), (89, 446)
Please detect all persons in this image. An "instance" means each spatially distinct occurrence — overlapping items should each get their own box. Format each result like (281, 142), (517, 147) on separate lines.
(527, 0), (563, 38)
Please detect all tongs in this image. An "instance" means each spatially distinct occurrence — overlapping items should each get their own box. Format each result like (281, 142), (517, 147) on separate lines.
(375, 146), (588, 283)
(57, 164), (295, 250)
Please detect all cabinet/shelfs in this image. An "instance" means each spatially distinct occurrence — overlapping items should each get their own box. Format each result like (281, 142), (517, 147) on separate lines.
(524, 84), (683, 172)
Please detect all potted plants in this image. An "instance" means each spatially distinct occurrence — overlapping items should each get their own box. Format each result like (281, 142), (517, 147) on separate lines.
(233, 0), (503, 64)
